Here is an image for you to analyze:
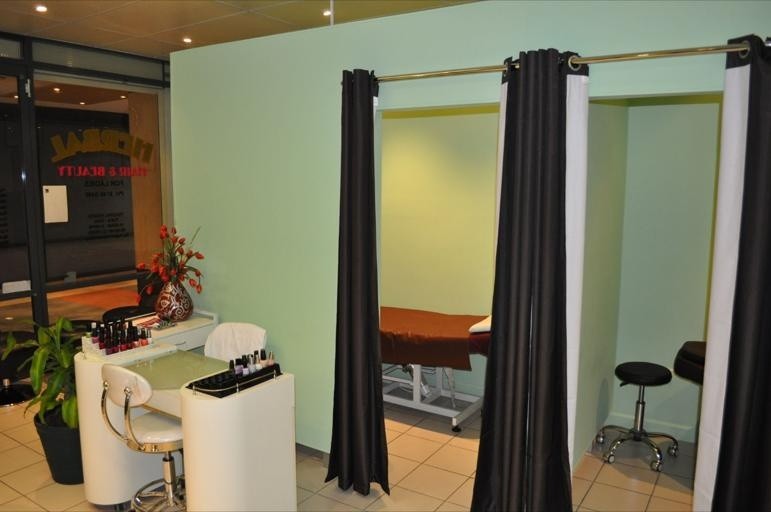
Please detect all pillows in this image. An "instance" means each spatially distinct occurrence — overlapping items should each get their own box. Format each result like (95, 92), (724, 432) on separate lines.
(467, 317), (490, 335)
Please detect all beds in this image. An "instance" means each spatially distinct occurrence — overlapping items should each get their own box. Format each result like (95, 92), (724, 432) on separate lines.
(379, 304), (496, 435)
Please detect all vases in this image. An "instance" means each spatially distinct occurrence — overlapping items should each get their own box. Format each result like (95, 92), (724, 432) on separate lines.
(155, 280), (195, 323)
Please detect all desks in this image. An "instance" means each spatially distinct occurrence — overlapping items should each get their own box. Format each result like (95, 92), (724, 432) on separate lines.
(74, 337), (298, 511)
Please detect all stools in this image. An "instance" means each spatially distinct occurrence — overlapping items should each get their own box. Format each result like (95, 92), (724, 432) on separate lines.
(593, 358), (680, 472)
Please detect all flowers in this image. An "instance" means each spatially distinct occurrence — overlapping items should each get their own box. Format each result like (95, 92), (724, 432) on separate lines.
(131, 226), (205, 294)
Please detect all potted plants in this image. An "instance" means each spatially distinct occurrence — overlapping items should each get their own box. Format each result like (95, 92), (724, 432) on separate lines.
(3, 315), (88, 485)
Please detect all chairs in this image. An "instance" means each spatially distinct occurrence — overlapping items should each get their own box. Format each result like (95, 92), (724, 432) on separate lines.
(98, 362), (186, 512)
